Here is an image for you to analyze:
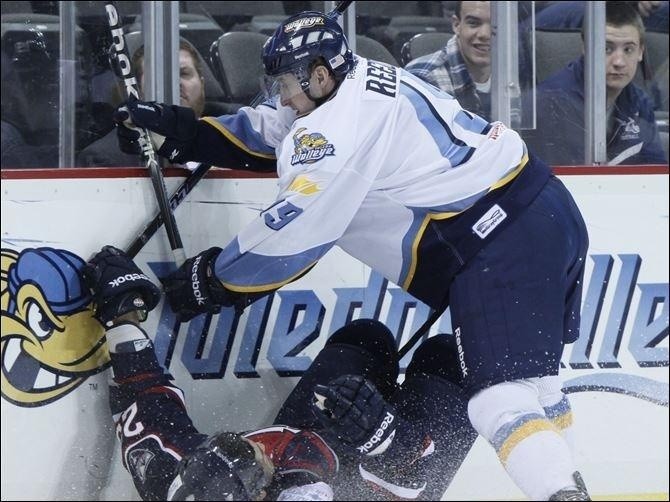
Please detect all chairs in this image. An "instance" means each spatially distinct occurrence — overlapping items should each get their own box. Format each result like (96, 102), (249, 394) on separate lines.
(0, 0), (670, 168)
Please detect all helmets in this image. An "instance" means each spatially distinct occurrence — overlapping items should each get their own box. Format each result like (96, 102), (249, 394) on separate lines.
(260, 8), (354, 86)
(166, 432), (268, 502)
(1, 28), (52, 64)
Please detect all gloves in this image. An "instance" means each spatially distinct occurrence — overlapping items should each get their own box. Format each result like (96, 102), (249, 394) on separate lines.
(116, 100), (198, 165)
(307, 373), (424, 472)
(77, 245), (160, 323)
(162, 247), (237, 323)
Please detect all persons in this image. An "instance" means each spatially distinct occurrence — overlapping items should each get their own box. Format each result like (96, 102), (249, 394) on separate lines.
(405, 0), (669, 167)
(77, 38), (209, 168)
(119, 12), (589, 501)
(79, 245), (478, 501)
